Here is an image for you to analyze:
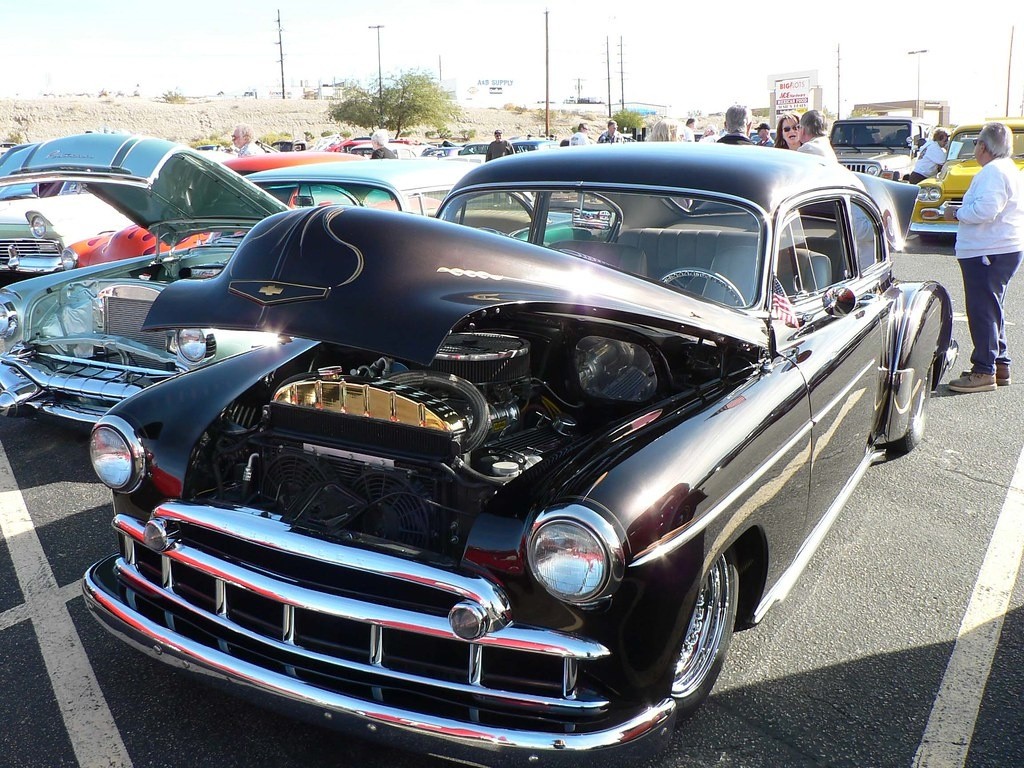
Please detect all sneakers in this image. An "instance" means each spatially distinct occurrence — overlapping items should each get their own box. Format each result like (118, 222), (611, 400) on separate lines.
(960, 365), (1011, 385)
(948, 373), (997, 392)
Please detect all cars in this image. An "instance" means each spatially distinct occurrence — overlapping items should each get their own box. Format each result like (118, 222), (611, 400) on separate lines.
(77, 141), (961, 768)
(1, 132), (605, 435)
(0, 127), (783, 285)
(908, 115), (1024, 244)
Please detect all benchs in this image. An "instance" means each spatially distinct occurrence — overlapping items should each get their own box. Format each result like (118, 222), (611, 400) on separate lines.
(617, 227), (844, 290)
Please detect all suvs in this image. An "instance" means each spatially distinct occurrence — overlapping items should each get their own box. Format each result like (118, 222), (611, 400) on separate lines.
(828, 113), (932, 183)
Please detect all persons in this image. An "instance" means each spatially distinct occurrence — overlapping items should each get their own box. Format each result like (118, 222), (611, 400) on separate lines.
(559, 139), (571, 194)
(644, 117), (692, 209)
(795, 110), (838, 162)
(484, 129), (516, 207)
(773, 110), (803, 151)
(550, 134), (555, 141)
(370, 129), (397, 159)
(231, 124), (266, 158)
(943, 122), (1024, 392)
(571, 122), (591, 202)
(753, 123), (775, 147)
(526, 134), (531, 140)
(910, 131), (947, 184)
(597, 120), (625, 143)
(698, 119), (728, 144)
(717, 104), (756, 145)
(678, 117), (695, 141)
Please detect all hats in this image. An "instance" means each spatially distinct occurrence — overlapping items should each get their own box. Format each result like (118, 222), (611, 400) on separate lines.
(494, 129), (502, 135)
(754, 124), (771, 130)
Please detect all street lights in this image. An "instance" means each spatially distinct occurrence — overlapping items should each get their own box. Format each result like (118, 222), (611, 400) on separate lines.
(909, 49), (928, 117)
(368, 25), (386, 129)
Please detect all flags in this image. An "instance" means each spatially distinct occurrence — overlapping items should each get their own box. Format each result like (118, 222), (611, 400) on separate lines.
(771, 277), (800, 329)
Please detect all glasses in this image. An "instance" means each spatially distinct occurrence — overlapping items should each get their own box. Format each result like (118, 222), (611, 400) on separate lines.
(783, 126), (800, 132)
(943, 139), (948, 142)
(231, 135), (249, 140)
(972, 139), (984, 145)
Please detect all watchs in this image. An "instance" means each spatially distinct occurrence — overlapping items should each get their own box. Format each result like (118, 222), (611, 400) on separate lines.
(952, 209), (959, 221)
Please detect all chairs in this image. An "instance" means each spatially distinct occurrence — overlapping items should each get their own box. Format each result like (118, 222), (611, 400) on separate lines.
(702, 246), (833, 308)
(550, 241), (646, 278)
